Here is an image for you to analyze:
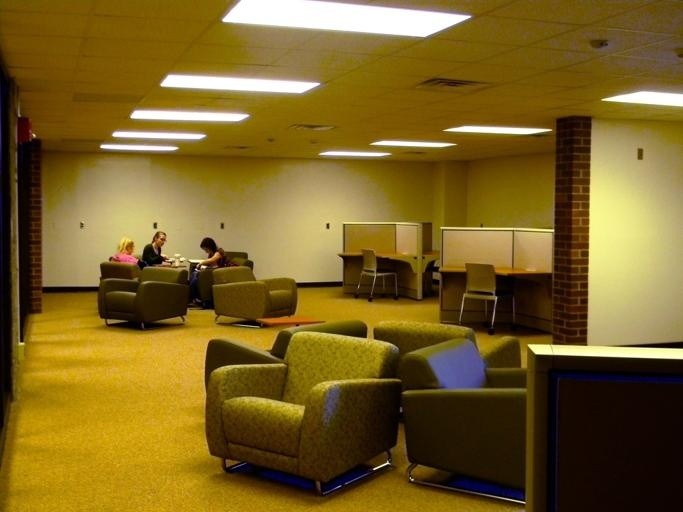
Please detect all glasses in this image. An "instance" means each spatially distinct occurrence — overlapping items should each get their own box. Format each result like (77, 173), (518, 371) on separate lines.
(160, 237), (166, 241)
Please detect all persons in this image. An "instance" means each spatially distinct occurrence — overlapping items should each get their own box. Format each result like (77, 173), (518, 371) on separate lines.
(186, 238), (231, 306)
(141, 232), (172, 269)
(110, 238), (141, 267)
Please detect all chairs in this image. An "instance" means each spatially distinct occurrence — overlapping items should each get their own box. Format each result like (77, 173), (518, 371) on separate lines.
(456, 263), (499, 335)
(354, 248), (399, 302)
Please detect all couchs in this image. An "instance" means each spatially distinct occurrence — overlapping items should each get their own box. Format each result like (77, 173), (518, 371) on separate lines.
(206, 330), (403, 497)
(373, 318), (522, 370)
(212, 267), (297, 327)
(402, 340), (528, 505)
(196, 257), (254, 310)
(99, 262), (144, 284)
(205, 321), (368, 390)
(96, 267), (190, 331)
(149, 258), (199, 306)
